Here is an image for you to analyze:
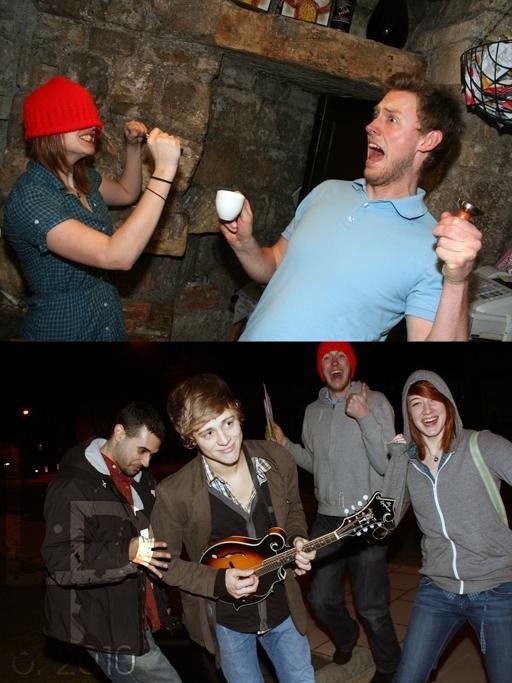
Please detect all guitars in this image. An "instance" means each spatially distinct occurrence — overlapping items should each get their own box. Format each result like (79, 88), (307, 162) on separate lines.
(197, 492), (397, 612)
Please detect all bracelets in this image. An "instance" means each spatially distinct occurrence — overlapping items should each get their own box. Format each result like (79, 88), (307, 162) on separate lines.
(145, 187), (167, 201)
(150, 175), (173, 185)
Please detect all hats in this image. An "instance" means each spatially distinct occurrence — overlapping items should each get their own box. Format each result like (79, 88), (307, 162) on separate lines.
(22, 73), (104, 141)
(316, 342), (357, 382)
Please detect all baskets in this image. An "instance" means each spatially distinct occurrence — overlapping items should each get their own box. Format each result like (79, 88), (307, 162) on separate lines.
(460, 38), (512, 129)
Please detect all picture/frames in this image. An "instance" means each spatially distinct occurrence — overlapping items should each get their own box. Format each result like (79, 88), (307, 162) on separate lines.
(275, 0), (336, 27)
(232, 0), (272, 13)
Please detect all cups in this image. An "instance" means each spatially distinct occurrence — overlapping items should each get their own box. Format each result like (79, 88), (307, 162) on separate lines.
(215, 188), (244, 220)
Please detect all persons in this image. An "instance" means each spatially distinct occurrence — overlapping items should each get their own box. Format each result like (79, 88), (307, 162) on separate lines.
(142, 372), (318, 683)
(0, 73), (183, 341)
(218, 70), (485, 342)
(264, 341), (402, 683)
(378, 368), (512, 682)
(37, 399), (184, 683)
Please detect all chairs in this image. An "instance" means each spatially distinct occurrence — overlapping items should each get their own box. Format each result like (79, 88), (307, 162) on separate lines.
(0, 416), (188, 585)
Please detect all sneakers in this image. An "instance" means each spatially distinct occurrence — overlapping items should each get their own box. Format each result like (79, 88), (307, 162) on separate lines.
(369, 670), (393, 683)
(333, 619), (359, 665)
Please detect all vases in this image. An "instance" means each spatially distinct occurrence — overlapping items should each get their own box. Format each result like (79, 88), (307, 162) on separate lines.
(366, 0), (409, 50)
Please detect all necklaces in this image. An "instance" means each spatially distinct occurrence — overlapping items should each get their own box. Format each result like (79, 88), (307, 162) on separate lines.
(426, 447), (442, 462)
